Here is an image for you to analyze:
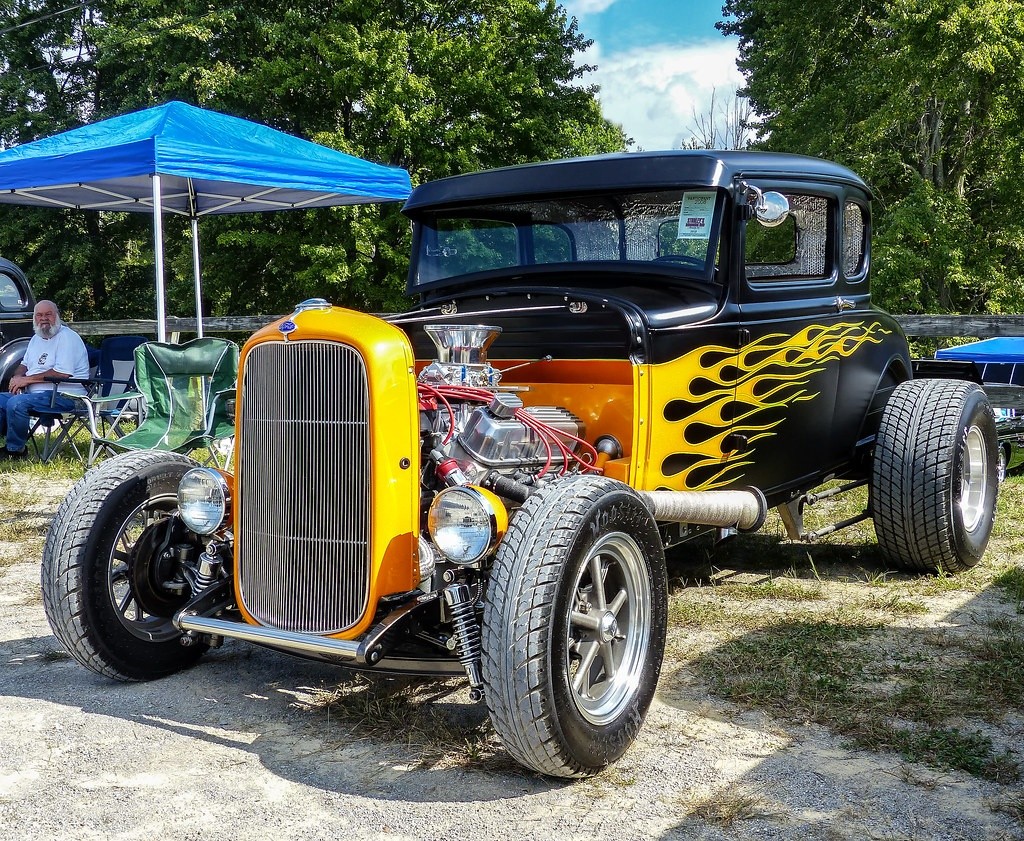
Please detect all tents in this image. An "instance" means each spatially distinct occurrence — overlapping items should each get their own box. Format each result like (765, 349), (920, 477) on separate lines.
(0, 101), (413, 350)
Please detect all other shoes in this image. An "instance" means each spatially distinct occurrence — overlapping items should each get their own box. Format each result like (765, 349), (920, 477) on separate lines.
(0, 444), (29, 462)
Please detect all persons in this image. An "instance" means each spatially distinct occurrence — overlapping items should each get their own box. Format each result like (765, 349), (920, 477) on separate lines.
(0, 299), (91, 460)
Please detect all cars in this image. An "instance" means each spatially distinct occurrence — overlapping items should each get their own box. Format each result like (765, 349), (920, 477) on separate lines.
(0, 258), (39, 395)
(40, 148), (1000, 780)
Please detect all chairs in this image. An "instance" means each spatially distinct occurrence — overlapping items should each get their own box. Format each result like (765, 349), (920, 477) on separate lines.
(21, 336), (240, 470)
